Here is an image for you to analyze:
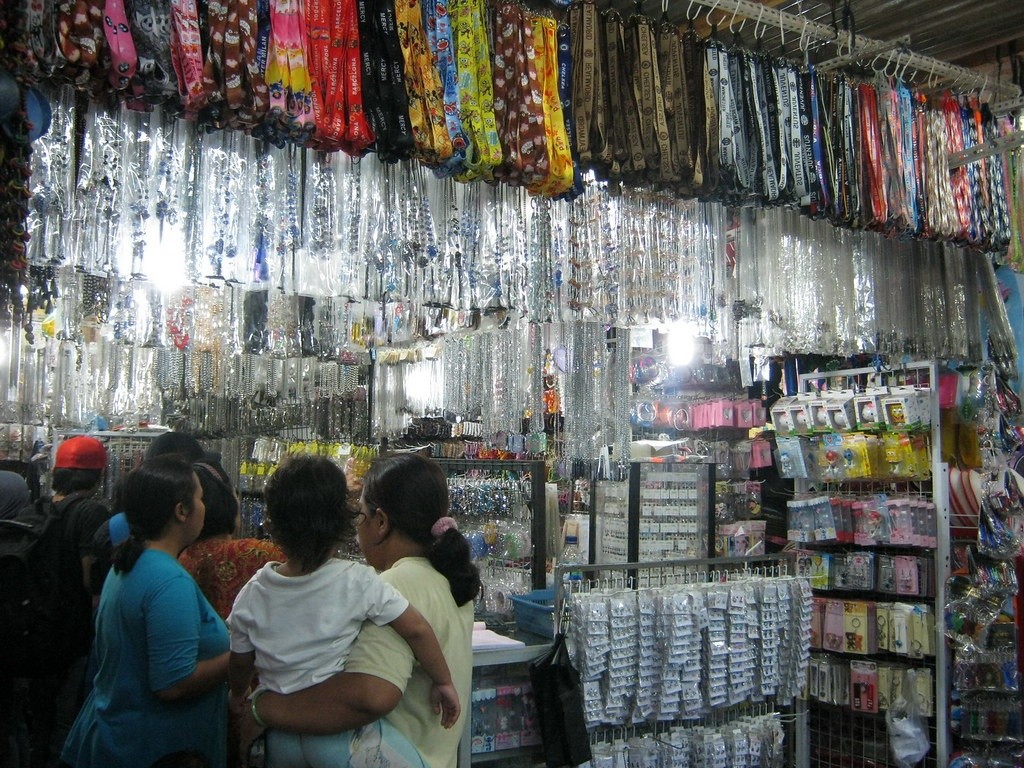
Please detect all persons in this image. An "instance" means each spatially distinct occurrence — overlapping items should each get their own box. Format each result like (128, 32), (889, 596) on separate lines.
(0, 427), (210, 767)
(227, 453), (461, 768)
(53, 453), (237, 768)
(172, 456), (294, 767)
(239, 453), (482, 768)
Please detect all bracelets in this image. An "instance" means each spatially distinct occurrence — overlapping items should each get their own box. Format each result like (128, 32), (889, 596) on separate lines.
(252, 688), (276, 729)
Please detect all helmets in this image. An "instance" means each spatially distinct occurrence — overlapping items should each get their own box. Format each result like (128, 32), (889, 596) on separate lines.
(56, 435), (108, 468)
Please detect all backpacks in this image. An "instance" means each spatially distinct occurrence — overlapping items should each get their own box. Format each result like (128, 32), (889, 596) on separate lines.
(0, 490), (90, 677)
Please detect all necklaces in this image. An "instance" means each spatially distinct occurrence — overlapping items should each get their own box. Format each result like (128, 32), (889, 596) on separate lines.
(1, 78), (1019, 478)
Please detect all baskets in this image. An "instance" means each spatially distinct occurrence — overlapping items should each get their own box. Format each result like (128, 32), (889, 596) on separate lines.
(507, 589), (573, 639)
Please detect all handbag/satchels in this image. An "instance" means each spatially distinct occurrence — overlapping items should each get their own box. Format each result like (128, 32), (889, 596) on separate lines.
(885, 669), (931, 768)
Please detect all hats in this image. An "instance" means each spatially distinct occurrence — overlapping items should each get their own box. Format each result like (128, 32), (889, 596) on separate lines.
(0, 470), (30, 519)
